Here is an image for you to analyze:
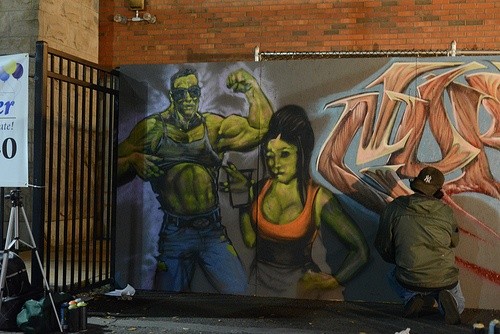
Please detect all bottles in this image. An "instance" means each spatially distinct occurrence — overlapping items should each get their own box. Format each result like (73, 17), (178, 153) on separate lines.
(489, 316), (500, 334)
(471, 320), (486, 334)
(60, 297), (68, 328)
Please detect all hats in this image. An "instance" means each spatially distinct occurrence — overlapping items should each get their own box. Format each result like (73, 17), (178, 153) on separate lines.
(410, 167), (445, 196)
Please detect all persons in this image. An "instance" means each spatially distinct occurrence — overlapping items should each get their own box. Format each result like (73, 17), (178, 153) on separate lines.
(375, 167), (466, 325)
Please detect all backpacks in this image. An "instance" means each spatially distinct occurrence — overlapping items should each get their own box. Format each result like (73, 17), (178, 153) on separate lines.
(0, 248), (32, 332)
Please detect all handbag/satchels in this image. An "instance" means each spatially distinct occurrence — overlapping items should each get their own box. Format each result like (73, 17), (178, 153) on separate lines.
(16, 291), (76, 334)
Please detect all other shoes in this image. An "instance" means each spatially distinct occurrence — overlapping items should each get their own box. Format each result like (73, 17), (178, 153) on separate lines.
(440, 291), (460, 324)
(405, 295), (425, 318)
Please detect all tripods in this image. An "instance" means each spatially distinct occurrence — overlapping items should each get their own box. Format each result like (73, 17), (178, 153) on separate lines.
(0, 189), (64, 334)
